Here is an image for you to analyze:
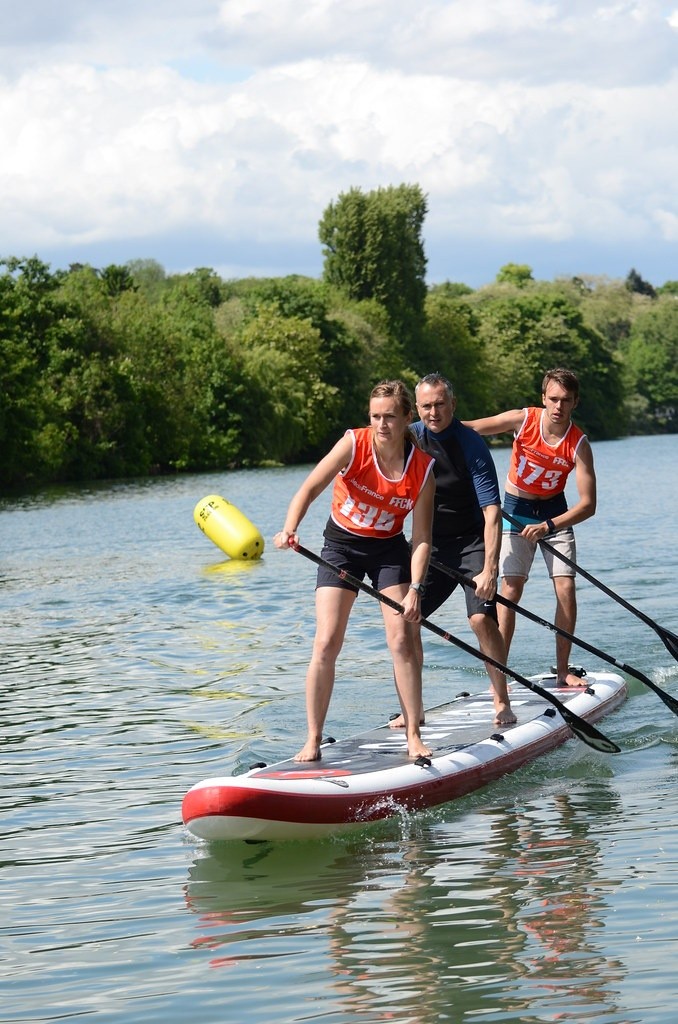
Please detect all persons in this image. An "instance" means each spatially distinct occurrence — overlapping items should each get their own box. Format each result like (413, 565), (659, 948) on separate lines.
(272, 382), (435, 757)
(458, 366), (597, 686)
(390, 371), (517, 726)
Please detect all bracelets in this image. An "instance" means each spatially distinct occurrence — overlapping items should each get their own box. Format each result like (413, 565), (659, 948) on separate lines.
(546, 518), (555, 533)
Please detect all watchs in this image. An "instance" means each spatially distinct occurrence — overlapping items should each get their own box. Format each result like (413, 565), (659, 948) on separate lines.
(408, 583), (426, 596)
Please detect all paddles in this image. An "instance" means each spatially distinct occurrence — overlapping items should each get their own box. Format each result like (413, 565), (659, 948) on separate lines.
(288, 536), (623, 755)
(501, 509), (678, 661)
(426, 555), (678, 715)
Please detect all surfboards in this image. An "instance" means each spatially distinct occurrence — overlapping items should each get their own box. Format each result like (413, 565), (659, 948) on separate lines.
(180, 673), (630, 844)
